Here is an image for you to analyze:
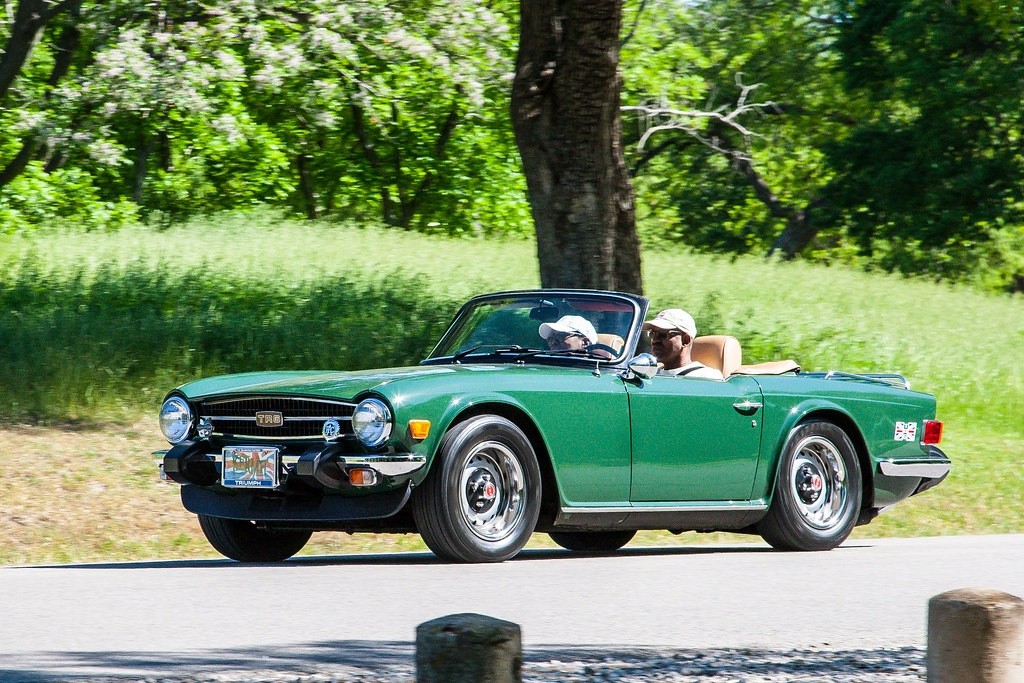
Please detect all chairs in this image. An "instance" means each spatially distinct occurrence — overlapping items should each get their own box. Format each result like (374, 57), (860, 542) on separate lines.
(591, 334), (624, 359)
(690, 335), (743, 379)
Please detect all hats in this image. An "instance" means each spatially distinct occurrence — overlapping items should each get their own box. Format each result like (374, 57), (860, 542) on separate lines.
(538, 315), (599, 346)
(643, 309), (697, 340)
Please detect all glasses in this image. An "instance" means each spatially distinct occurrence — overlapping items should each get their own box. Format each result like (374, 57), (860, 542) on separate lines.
(547, 331), (583, 348)
(646, 328), (684, 340)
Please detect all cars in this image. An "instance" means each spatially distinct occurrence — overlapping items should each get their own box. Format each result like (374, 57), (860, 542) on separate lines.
(151, 289), (952, 563)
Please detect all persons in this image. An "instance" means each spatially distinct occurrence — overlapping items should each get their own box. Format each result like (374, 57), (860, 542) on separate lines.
(643, 308), (726, 383)
(538, 314), (599, 355)
